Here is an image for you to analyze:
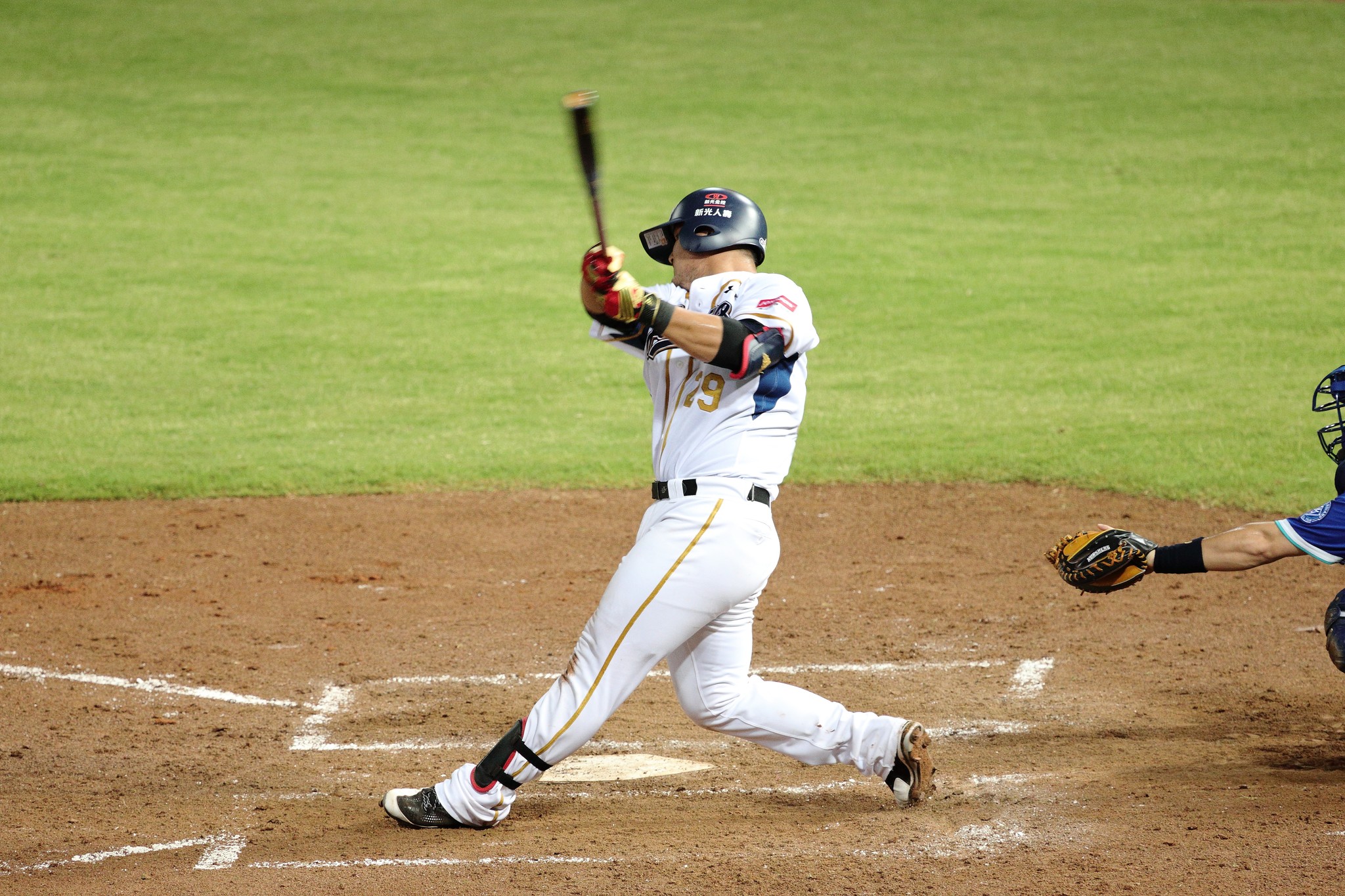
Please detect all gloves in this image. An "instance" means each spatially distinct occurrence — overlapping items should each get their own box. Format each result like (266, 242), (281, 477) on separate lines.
(582, 242), (624, 293)
(594, 270), (675, 335)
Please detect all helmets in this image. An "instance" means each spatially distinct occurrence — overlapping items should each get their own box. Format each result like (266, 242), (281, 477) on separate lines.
(638, 188), (766, 267)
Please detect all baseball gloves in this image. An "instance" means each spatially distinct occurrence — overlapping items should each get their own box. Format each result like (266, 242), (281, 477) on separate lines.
(1047, 524), (1161, 596)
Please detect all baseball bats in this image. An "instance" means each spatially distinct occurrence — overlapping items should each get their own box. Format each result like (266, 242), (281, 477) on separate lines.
(565, 92), (629, 338)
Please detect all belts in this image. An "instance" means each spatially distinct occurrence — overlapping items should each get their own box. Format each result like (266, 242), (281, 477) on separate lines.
(650, 478), (770, 505)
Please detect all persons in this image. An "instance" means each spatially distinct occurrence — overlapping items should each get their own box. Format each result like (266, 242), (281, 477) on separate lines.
(383, 187), (939, 826)
(1049, 365), (1345, 673)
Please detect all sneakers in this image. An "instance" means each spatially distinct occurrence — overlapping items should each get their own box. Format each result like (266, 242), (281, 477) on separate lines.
(885, 722), (935, 807)
(378, 786), (468, 829)
